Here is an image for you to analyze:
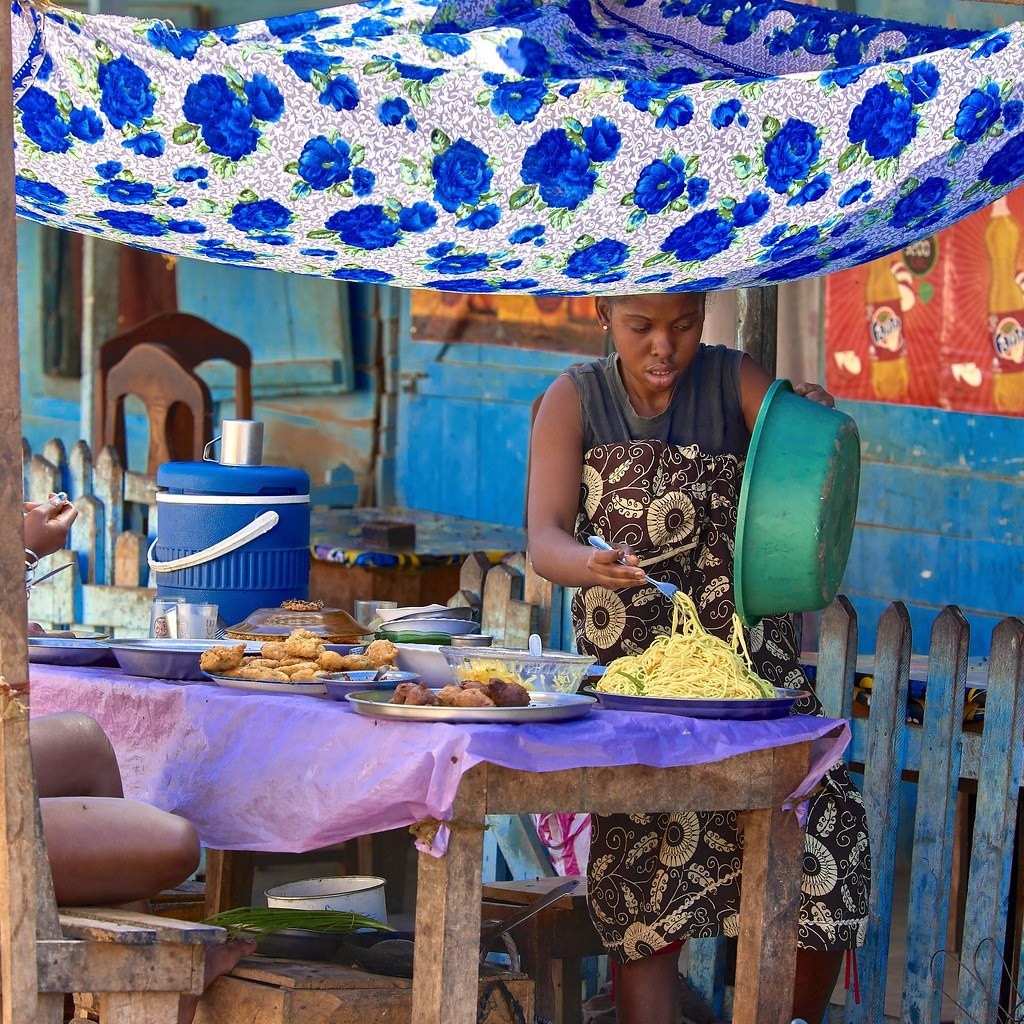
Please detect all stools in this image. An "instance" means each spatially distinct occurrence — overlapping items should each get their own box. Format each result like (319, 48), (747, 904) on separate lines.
(480, 875), (609, 1024)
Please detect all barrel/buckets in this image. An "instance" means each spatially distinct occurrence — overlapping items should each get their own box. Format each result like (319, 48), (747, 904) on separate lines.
(264, 873), (388, 926)
(145, 459), (311, 627)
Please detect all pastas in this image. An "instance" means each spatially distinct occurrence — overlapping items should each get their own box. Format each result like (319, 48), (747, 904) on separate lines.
(595, 591), (779, 700)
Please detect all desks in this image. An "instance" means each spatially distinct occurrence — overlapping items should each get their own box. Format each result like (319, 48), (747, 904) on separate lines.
(27, 662), (853, 1024)
(308, 506), (529, 618)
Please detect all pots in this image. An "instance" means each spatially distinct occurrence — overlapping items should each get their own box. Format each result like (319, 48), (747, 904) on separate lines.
(343, 879), (580, 978)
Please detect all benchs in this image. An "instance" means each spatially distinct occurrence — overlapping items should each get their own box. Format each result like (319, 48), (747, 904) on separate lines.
(29, 751), (229, 1024)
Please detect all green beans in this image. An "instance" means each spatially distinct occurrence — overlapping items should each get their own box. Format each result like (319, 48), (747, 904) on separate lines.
(615, 635), (767, 700)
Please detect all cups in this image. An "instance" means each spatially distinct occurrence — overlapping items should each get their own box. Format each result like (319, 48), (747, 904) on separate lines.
(202, 418), (264, 467)
(149, 595), (186, 639)
(175, 603), (218, 640)
(355, 599), (397, 628)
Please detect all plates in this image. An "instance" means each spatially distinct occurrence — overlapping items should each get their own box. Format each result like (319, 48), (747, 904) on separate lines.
(345, 687), (599, 724)
(221, 597), (482, 656)
(201, 669), (327, 693)
(95, 638), (271, 681)
(26, 637), (111, 667)
(583, 686), (811, 720)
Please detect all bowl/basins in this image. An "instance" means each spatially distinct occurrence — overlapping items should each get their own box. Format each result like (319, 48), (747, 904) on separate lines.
(734, 377), (861, 629)
(226, 921), (340, 961)
(393, 642), (598, 695)
(316, 669), (420, 701)
(449, 632), (492, 647)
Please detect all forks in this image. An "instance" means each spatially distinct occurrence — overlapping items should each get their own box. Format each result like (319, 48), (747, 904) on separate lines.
(588, 535), (680, 601)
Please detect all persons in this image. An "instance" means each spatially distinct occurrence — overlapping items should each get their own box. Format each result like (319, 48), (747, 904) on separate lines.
(528, 290), (872, 1024)
(22, 492), (201, 906)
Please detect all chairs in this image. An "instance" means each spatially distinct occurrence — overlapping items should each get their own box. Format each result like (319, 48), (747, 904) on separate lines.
(103, 345), (216, 478)
(91, 309), (253, 467)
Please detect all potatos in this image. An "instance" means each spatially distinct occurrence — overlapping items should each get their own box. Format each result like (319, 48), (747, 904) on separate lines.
(387, 679), (530, 708)
